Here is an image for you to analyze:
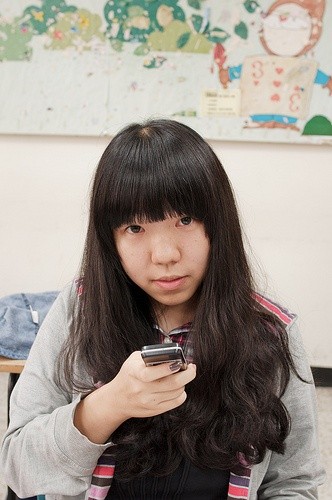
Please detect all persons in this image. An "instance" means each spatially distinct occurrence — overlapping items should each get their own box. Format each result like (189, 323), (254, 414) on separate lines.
(0, 119), (328, 500)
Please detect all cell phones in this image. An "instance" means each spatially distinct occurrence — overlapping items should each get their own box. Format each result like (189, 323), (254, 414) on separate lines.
(141, 343), (187, 375)
